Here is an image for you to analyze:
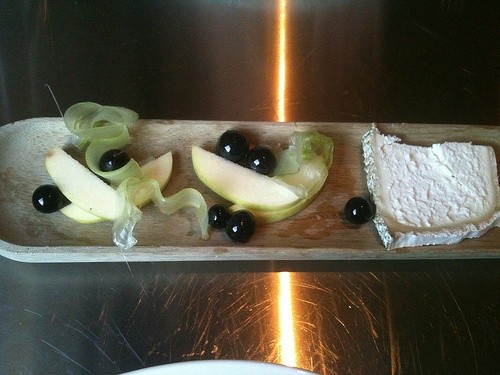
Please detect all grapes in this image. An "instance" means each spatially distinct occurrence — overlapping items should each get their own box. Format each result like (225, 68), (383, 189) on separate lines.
(30, 129), (376, 245)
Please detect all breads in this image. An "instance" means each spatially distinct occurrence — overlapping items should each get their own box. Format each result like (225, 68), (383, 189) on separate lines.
(360, 125), (500, 249)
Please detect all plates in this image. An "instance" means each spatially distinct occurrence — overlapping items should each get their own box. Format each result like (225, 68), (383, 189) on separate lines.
(0, 116), (499, 263)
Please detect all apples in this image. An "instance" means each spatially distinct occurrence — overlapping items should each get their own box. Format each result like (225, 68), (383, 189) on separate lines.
(44, 144), (333, 226)
